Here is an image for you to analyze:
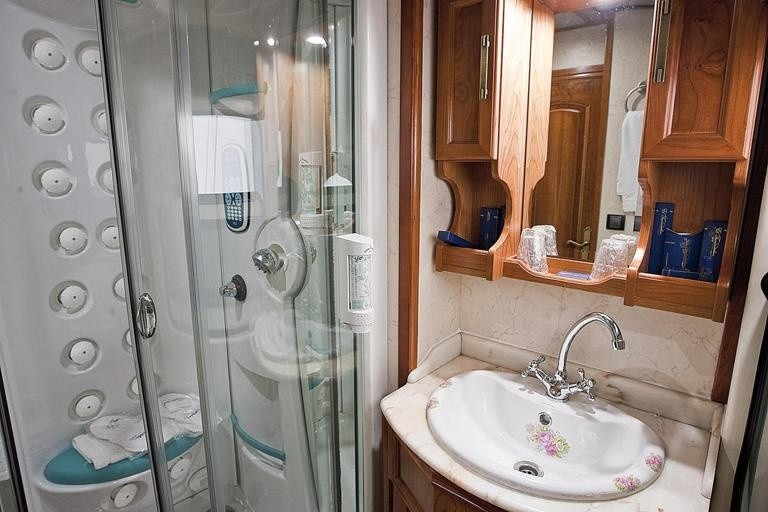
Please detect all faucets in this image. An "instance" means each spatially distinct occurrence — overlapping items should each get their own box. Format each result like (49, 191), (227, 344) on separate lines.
(545, 311), (626, 401)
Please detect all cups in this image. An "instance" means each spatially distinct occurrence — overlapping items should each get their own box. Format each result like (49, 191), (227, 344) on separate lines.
(610, 233), (636, 264)
(520, 228), (547, 275)
(534, 225), (559, 258)
(589, 240), (627, 280)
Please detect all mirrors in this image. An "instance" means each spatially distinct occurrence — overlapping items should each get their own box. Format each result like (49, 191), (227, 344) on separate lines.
(523, 2), (655, 298)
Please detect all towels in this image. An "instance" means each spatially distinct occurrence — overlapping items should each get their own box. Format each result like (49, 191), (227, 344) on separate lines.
(613, 109), (646, 215)
(67, 394), (208, 471)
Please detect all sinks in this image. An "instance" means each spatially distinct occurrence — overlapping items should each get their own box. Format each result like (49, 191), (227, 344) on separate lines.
(425, 370), (665, 501)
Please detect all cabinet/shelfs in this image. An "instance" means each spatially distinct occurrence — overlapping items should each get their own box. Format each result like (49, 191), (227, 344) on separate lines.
(623, 1), (767, 322)
(434, 1), (528, 282)
(520, 2), (556, 255)
(381, 412), (508, 510)
